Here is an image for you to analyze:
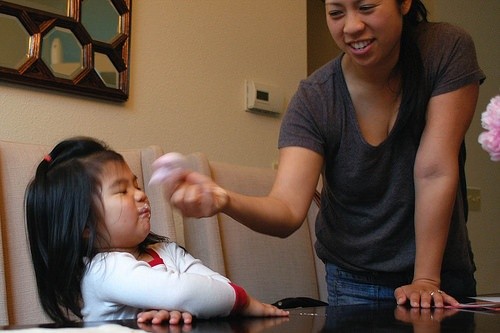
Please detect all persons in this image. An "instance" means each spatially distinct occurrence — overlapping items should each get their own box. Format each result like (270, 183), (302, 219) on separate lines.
(23, 136), (290, 324)
(164, 0), (487, 309)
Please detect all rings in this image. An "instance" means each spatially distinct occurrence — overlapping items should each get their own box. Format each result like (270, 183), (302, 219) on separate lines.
(430, 289), (443, 297)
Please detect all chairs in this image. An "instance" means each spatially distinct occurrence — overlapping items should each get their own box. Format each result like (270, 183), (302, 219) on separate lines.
(0, 140), (177, 330)
(183, 152), (328, 304)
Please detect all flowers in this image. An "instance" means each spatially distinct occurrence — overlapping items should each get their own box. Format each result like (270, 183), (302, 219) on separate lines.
(477, 95), (500, 161)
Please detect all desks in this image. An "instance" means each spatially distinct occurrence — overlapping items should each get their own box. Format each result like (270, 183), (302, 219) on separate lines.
(0, 292), (500, 333)
